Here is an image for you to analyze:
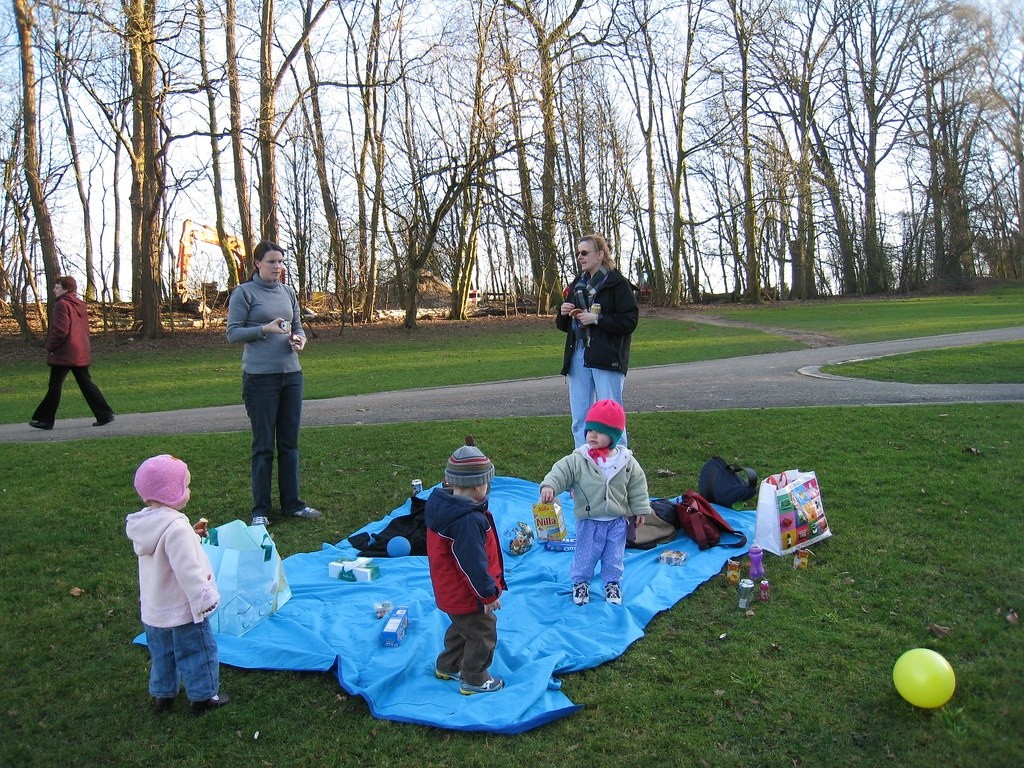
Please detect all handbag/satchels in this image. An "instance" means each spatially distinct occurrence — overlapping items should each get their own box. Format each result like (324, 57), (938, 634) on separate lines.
(676, 490), (747, 549)
(625, 507), (676, 549)
(754, 468), (832, 557)
(700, 455), (757, 508)
(200, 519), (292, 639)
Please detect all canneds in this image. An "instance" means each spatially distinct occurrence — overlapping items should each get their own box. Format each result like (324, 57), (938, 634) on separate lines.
(412, 479), (422, 495)
(591, 303), (601, 313)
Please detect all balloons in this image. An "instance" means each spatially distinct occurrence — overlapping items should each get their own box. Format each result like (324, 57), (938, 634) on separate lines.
(500, 521), (534, 555)
(387, 536), (411, 556)
(893, 648), (956, 708)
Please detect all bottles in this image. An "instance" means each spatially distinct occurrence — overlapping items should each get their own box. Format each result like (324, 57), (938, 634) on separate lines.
(749, 540), (765, 578)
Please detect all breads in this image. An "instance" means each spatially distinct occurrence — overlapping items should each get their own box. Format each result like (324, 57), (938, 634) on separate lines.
(197, 518), (208, 537)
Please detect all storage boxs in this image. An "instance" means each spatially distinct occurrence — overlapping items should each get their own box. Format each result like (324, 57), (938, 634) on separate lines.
(533, 497), (568, 541)
(380, 607), (409, 647)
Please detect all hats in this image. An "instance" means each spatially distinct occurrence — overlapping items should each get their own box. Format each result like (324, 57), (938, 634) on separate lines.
(134, 455), (187, 507)
(444, 446), (495, 487)
(584, 400), (625, 465)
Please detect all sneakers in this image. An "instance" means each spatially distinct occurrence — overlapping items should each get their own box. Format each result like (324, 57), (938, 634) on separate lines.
(435, 668), (462, 681)
(573, 581), (589, 605)
(251, 517), (270, 529)
(604, 581), (622, 604)
(459, 677), (503, 695)
(289, 507), (322, 518)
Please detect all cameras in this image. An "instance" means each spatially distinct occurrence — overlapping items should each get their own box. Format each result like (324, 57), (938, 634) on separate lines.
(281, 321), (291, 331)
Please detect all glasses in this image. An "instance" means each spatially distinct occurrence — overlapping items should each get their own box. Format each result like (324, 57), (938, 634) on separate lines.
(576, 250), (598, 256)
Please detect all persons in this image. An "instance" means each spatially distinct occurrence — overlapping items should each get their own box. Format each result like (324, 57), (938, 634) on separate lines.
(425, 446), (508, 695)
(126, 455), (230, 716)
(556, 236), (639, 449)
(540, 400), (651, 606)
(227, 241), (321, 529)
(29, 276), (115, 430)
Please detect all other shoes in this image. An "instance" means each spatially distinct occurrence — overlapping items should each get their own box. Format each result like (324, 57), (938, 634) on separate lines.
(93, 414), (115, 426)
(153, 698), (174, 715)
(29, 420), (52, 429)
(188, 694), (229, 715)
